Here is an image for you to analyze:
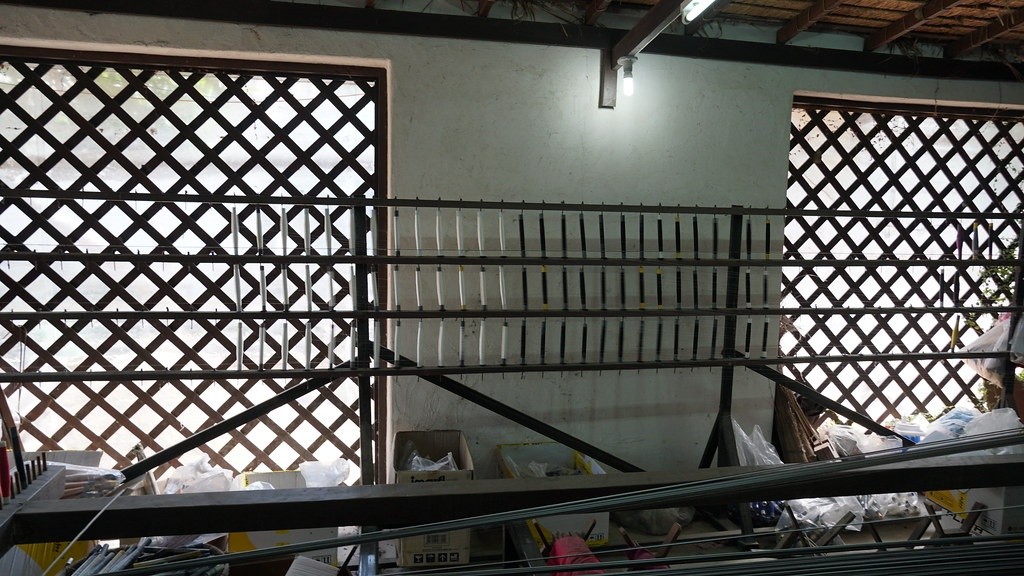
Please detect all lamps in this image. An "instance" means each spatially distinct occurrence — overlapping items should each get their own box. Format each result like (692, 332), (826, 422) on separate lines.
(680, 0), (715, 25)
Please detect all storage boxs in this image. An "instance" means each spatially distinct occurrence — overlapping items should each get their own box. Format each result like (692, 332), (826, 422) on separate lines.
(0, 449), (103, 576)
(908, 431), (1024, 537)
(388, 427), (473, 567)
(497, 441), (610, 550)
(224, 458), (361, 568)
